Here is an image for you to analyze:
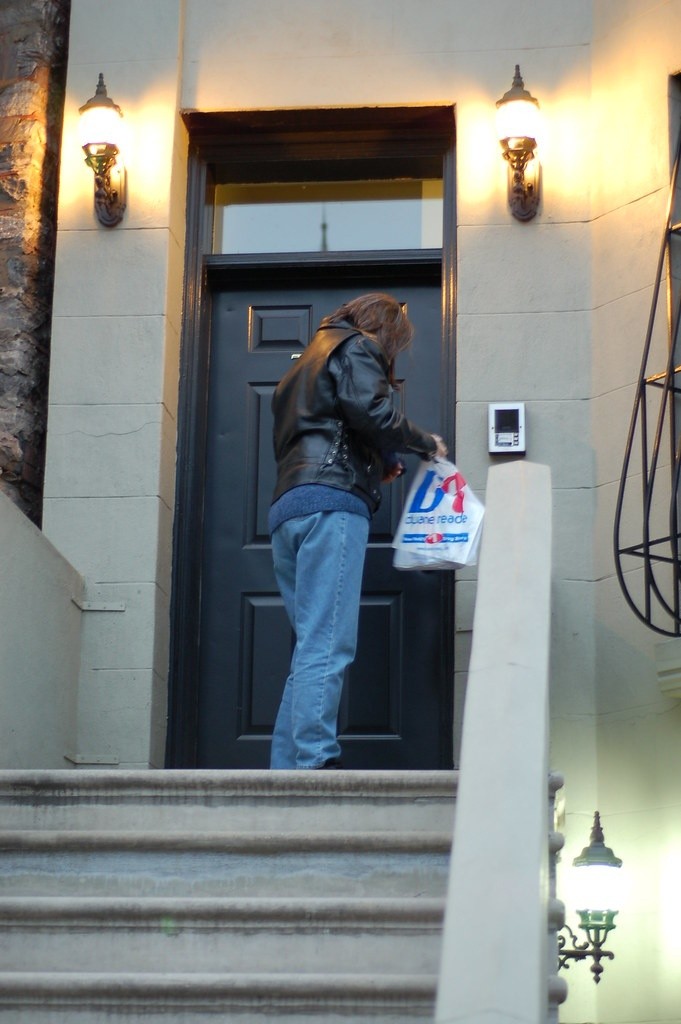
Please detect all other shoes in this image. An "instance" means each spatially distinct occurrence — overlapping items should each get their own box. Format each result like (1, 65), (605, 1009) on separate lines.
(318, 758), (343, 769)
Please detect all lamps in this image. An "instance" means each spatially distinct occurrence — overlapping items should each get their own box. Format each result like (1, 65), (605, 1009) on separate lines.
(78, 73), (126, 228)
(495, 64), (542, 223)
(557, 808), (622, 985)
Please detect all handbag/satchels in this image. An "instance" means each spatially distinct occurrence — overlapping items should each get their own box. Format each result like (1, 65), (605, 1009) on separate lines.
(391, 455), (486, 571)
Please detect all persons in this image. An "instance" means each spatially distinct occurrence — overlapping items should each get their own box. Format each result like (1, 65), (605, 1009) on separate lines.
(270, 291), (445, 771)
